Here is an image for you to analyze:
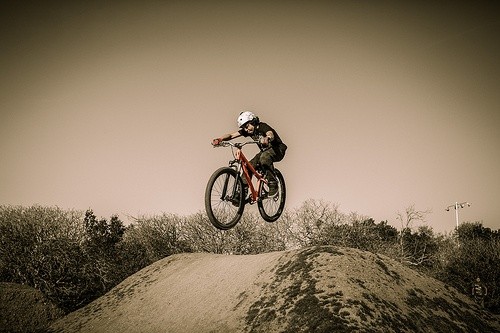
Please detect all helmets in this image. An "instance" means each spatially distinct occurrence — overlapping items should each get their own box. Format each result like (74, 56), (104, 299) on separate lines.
(237, 111), (256, 128)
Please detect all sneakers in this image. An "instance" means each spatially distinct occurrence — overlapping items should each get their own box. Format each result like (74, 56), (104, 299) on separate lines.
(229, 194), (240, 201)
(268, 183), (279, 197)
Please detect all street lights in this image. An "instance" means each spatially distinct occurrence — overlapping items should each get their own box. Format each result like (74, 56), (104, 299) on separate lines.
(446, 201), (471, 249)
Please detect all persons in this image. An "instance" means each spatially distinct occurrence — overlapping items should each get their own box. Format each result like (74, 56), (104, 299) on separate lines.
(212, 111), (288, 207)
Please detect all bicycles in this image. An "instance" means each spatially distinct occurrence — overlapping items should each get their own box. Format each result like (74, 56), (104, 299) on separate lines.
(204, 141), (286, 231)
